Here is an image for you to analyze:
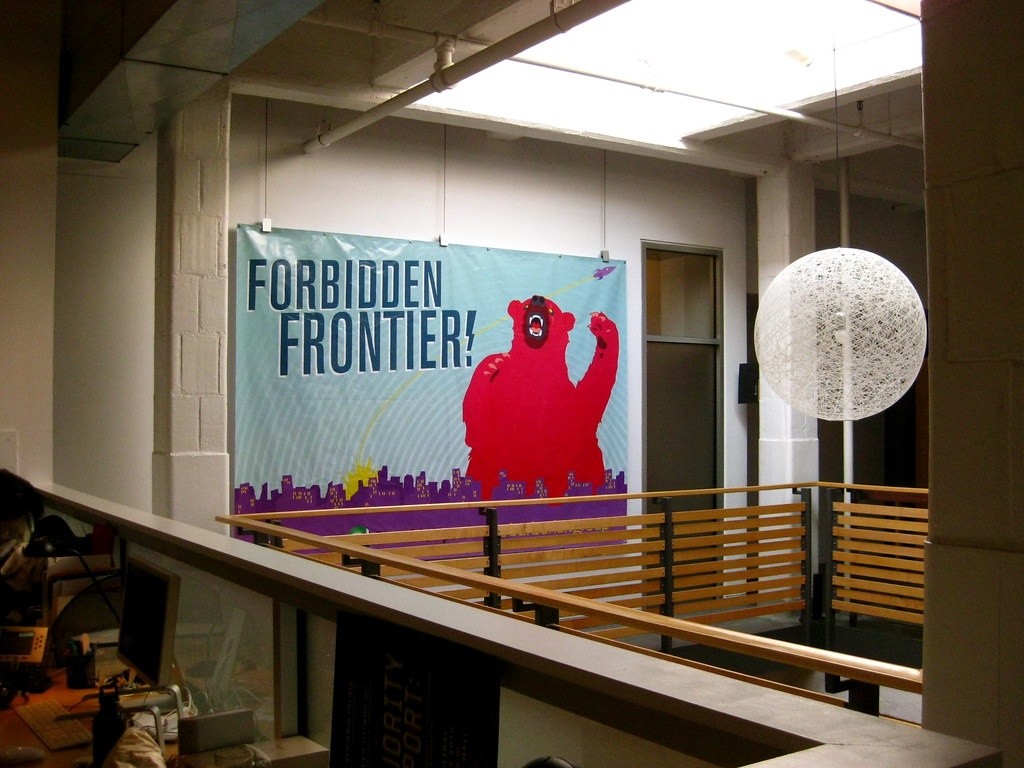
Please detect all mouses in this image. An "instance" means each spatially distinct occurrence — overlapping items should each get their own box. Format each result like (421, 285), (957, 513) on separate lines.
(0, 746), (45, 767)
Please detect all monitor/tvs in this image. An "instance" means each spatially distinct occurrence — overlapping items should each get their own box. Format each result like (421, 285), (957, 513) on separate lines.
(117, 553), (181, 690)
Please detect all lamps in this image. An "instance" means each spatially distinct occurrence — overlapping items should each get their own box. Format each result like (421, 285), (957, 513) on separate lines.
(21, 515), (121, 560)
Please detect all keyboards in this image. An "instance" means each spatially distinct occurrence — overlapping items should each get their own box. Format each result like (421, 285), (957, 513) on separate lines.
(15, 697), (93, 752)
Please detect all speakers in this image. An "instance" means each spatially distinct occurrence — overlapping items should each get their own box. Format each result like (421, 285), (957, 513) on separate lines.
(738, 363), (760, 404)
